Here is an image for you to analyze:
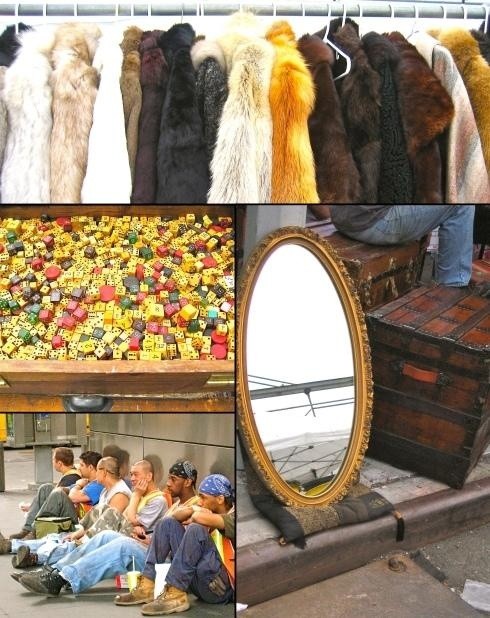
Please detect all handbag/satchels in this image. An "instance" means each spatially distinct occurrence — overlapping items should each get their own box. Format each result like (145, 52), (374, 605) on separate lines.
(35, 515), (70, 540)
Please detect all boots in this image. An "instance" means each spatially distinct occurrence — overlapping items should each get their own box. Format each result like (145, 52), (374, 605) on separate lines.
(114, 575), (155, 605)
(142, 583), (191, 616)
(12, 545), (38, 568)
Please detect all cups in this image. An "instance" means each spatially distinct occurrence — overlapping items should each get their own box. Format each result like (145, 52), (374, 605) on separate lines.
(126, 571), (142, 594)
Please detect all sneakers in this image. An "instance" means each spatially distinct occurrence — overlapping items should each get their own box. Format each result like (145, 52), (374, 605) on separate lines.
(11, 566), (63, 598)
(10, 528), (36, 540)
(0, 532), (10, 554)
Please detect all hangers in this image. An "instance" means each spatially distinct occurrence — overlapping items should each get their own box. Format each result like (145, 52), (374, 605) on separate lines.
(14, 2), (490, 81)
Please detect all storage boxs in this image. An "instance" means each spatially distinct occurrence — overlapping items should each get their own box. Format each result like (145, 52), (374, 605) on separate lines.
(308, 219), (490, 489)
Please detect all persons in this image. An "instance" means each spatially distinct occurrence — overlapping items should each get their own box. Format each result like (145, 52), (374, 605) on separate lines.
(75, 460), (173, 547)
(9, 450), (105, 541)
(9, 461), (199, 596)
(329, 203), (477, 289)
(115, 474), (236, 616)
(19, 446), (82, 512)
(1, 456), (131, 569)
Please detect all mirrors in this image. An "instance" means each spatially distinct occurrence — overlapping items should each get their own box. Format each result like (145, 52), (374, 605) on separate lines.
(236, 226), (375, 508)
